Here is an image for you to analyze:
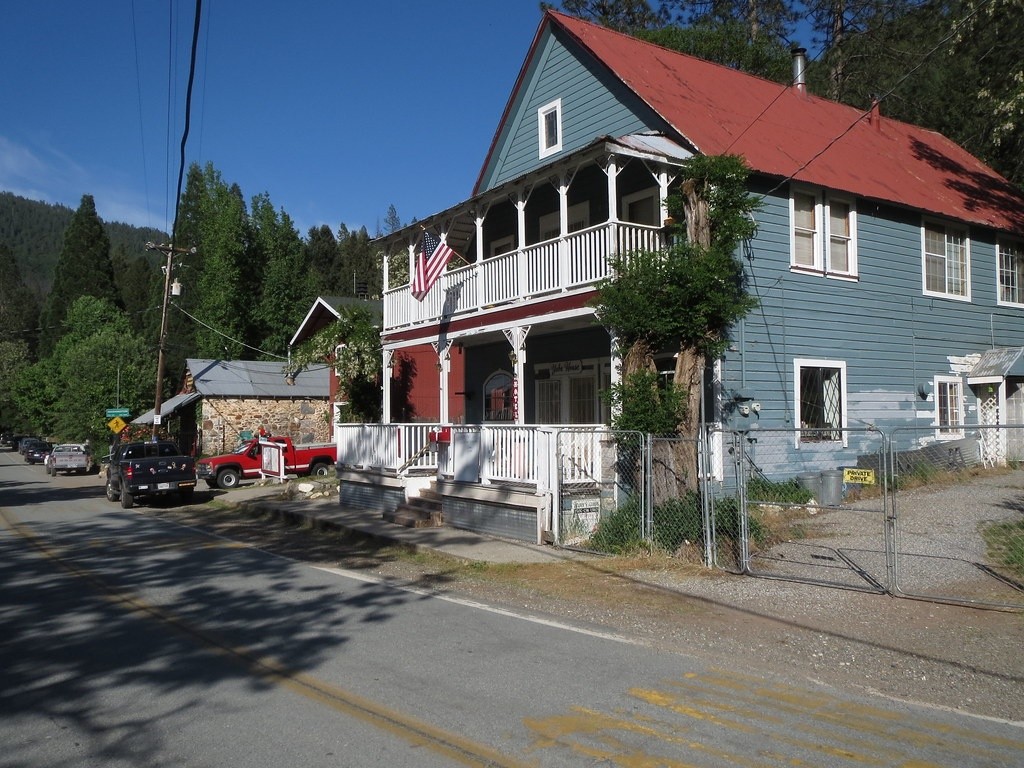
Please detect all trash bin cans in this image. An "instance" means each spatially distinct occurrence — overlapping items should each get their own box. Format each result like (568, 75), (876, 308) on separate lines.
(796, 472), (821, 503)
(821, 471), (843, 506)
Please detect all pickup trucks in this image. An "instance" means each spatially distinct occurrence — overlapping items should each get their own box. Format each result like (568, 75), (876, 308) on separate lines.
(47, 445), (88, 477)
(106, 441), (197, 509)
(197, 427), (337, 489)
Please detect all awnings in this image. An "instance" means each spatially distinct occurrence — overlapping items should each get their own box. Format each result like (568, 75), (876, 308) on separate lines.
(129, 393), (200, 426)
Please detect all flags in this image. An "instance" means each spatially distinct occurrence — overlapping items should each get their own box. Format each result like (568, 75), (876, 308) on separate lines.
(411, 231), (456, 303)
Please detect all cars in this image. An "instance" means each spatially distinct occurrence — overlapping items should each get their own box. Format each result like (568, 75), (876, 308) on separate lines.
(13, 437), (56, 465)
